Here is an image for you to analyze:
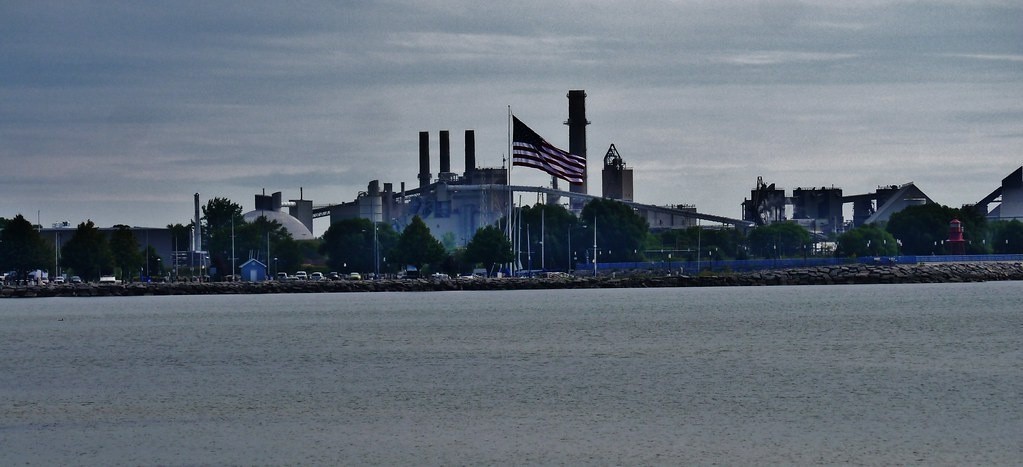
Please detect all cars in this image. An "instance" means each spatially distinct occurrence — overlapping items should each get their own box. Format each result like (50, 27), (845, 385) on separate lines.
(54, 277), (66, 284)
(69, 275), (82, 283)
(311, 272), (325, 281)
(295, 271), (308, 280)
(350, 272), (361, 280)
(329, 272), (342, 281)
(277, 272), (288, 280)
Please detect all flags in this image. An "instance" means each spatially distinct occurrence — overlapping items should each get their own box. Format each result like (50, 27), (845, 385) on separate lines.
(513, 115), (586, 186)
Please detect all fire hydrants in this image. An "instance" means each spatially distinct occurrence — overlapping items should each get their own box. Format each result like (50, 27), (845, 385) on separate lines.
(946, 219), (965, 257)
